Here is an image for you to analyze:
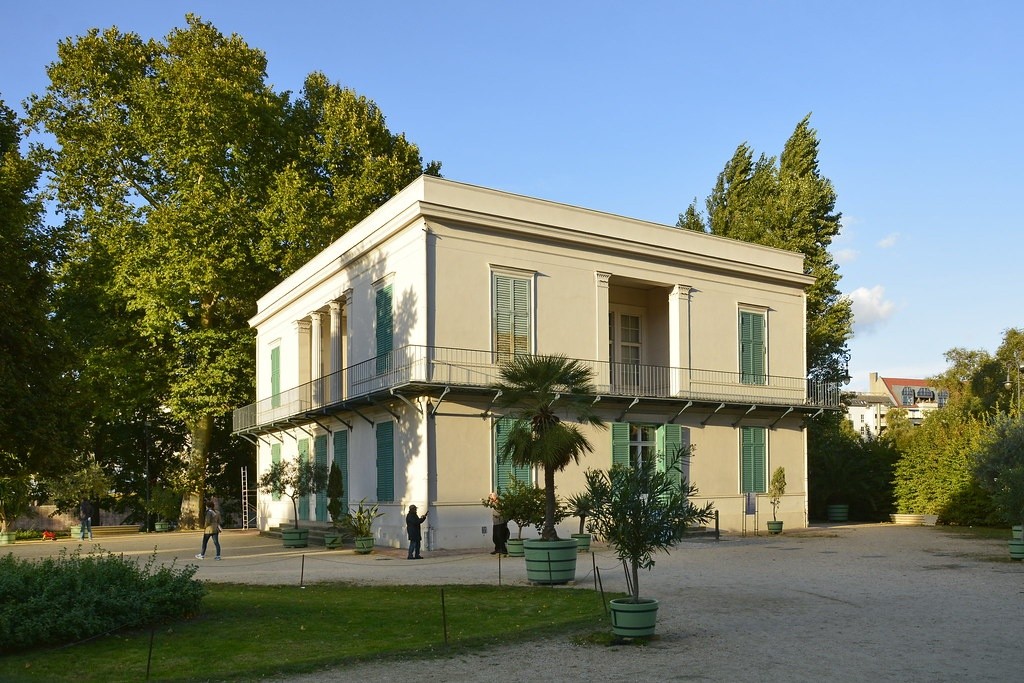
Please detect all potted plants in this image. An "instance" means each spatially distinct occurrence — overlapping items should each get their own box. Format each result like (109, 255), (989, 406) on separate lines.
(250, 455), (385, 553)
(481, 352), (721, 640)
(0, 477), (32, 543)
(145, 488), (178, 532)
(980, 463), (1024, 559)
(766, 466), (786, 532)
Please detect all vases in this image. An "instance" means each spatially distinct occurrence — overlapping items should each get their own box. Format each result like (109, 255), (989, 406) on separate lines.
(827, 505), (848, 520)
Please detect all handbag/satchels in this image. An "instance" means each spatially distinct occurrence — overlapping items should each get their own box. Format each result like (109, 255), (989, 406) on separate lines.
(216, 525), (222, 533)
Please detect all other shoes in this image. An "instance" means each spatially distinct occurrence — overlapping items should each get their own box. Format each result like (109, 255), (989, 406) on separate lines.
(214, 556), (221, 560)
(407, 556), (415, 559)
(194, 554), (204, 560)
(489, 551), (499, 556)
(415, 555), (423, 559)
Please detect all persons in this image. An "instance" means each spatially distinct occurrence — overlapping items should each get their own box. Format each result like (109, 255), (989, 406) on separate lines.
(79, 497), (94, 541)
(487, 494), (509, 557)
(406, 505), (428, 560)
(194, 501), (222, 561)
